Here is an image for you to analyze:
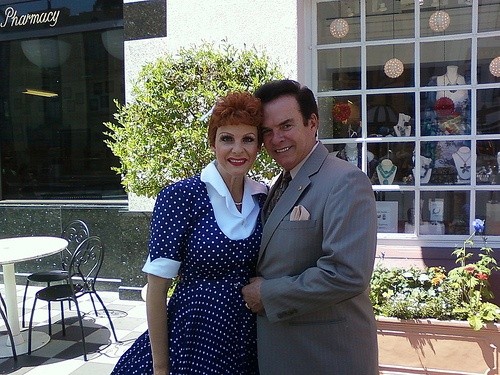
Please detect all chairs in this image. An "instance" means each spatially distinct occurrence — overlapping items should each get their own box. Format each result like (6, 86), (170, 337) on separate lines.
(28, 236), (116, 362)
(21, 219), (98, 328)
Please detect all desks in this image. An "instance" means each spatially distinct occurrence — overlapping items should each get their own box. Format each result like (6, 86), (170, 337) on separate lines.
(0, 236), (68, 360)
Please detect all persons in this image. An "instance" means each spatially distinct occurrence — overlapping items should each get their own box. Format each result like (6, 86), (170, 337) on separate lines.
(110, 92), (271, 375)
(242, 79), (379, 375)
(425, 66), (471, 136)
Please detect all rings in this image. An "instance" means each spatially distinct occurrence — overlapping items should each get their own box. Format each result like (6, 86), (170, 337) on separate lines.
(245, 302), (250, 309)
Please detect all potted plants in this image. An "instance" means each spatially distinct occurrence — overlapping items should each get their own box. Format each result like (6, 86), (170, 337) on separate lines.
(102, 35), (295, 308)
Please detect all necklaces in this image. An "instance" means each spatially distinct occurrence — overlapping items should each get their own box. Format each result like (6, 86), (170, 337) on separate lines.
(455, 152), (471, 173)
(446, 74), (460, 93)
(378, 164), (396, 185)
(345, 147), (358, 162)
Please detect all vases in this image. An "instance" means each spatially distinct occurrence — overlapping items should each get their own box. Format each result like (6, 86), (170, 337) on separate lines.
(374, 316), (500, 375)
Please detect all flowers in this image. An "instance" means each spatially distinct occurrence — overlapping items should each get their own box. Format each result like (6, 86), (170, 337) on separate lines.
(368, 218), (500, 331)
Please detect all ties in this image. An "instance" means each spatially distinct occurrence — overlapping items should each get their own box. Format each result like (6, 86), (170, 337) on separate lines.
(264, 171), (292, 221)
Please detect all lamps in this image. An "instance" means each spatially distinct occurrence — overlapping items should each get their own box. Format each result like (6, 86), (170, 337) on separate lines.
(429, 0), (450, 32)
(383, 0), (403, 78)
(489, 56), (500, 77)
(367, 105), (399, 158)
(332, 38), (351, 121)
(433, 5), (454, 117)
(330, 0), (348, 39)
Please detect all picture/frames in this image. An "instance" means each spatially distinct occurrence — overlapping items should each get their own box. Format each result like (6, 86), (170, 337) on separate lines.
(63, 87), (84, 112)
(88, 81), (114, 110)
